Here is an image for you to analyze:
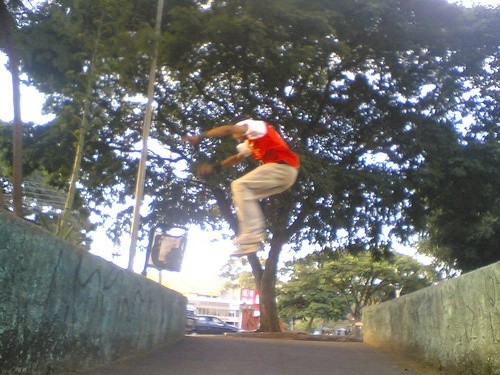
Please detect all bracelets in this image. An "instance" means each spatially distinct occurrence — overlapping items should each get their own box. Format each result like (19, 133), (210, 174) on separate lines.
(211, 162), (221, 173)
(198, 131), (205, 141)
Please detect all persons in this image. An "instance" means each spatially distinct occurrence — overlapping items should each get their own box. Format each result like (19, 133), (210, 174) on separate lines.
(183, 117), (300, 258)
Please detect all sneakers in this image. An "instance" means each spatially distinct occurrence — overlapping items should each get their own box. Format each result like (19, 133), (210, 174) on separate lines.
(231, 243), (262, 257)
(233, 230), (262, 244)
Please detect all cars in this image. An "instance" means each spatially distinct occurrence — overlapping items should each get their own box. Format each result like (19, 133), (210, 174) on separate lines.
(193, 314), (239, 337)
